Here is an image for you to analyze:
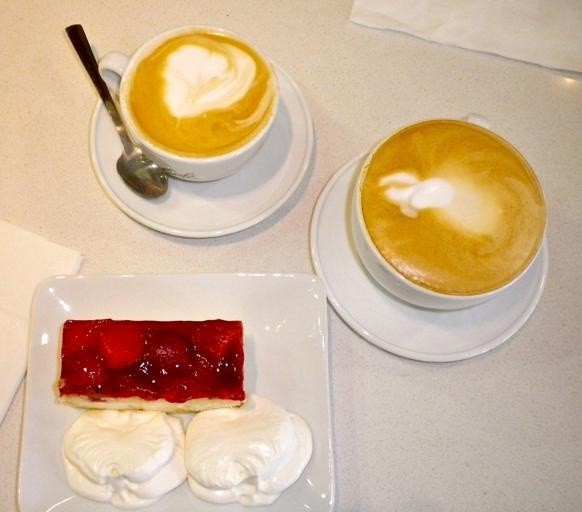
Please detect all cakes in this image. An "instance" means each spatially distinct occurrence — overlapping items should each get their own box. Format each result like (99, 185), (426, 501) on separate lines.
(52, 317), (248, 411)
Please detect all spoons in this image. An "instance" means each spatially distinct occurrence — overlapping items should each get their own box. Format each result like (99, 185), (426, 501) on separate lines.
(66, 24), (168, 199)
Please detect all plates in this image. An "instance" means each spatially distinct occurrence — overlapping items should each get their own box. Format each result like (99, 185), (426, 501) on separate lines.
(90, 56), (313, 239)
(18, 273), (336, 512)
(309, 149), (549, 362)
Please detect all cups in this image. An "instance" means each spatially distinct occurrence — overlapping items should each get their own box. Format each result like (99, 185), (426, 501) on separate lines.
(351, 113), (548, 310)
(98, 25), (280, 182)
